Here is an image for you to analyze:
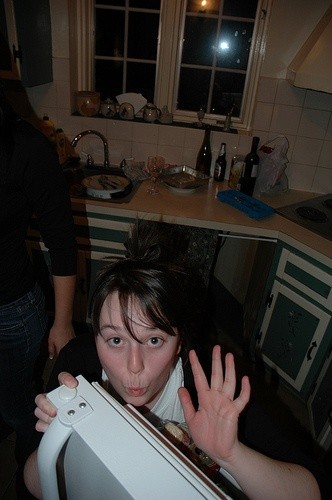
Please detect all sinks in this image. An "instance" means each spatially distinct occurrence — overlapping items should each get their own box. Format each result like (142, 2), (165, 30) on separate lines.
(61, 167), (145, 204)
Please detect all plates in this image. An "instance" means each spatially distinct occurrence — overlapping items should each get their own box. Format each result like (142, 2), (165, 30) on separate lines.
(158, 165), (211, 193)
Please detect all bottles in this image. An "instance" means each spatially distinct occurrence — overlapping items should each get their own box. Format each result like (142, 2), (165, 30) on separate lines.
(37, 112), (54, 143)
(196, 126), (212, 176)
(240, 137), (260, 197)
(214, 143), (227, 181)
(56, 128), (65, 164)
(229, 145), (244, 188)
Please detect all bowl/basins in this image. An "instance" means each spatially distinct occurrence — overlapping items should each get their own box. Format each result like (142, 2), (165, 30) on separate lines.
(76, 91), (100, 116)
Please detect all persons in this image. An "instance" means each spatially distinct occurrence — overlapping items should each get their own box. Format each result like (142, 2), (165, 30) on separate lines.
(17, 222), (332, 500)
(0, 87), (78, 500)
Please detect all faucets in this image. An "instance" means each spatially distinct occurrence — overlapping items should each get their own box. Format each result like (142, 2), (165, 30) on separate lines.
(70, 129), (109, 170)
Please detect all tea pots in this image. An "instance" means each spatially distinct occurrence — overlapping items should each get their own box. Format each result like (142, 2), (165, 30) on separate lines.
(101, 100), (118, 117)
(143, 103), (161, 123)
(119, 103), (137, 120)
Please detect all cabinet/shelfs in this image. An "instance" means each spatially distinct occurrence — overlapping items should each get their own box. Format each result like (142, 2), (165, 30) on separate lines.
(69, 205), (332, 459)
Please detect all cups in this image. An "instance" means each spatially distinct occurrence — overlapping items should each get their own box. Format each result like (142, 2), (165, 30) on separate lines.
(197, 105), (207, 127)
